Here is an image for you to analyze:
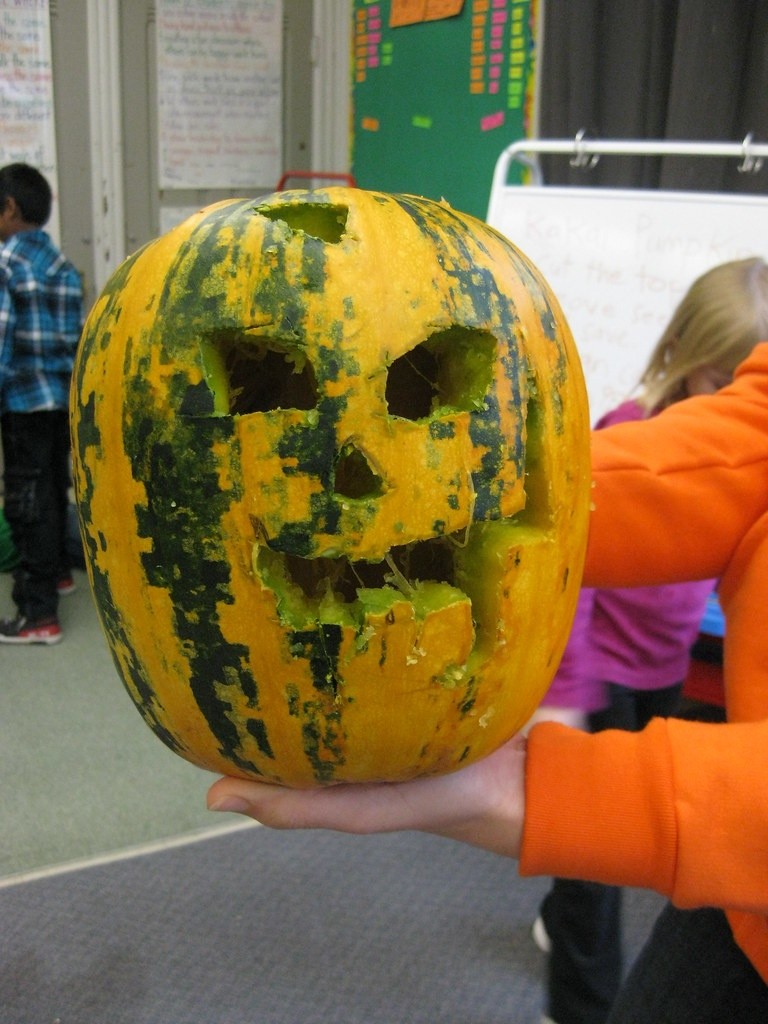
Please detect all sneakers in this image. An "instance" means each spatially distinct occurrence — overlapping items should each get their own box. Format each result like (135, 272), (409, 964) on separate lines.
(51, 576), (72, 599)
(2, 615), (66, 647)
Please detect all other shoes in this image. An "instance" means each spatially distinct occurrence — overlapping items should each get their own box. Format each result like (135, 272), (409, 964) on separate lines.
(532, 895), (553, 953)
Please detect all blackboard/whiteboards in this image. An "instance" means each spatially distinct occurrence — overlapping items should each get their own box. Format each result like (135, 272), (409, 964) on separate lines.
(484, 129), (768, 432)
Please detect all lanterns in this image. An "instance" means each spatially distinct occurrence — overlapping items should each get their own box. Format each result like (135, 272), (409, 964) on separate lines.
(69, 179), (596, 797)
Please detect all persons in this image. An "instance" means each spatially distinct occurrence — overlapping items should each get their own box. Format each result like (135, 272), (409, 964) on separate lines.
(204, 332), (768, 1024)
(529, 253), (768, 1024)
(1, 161), (84, 651)
